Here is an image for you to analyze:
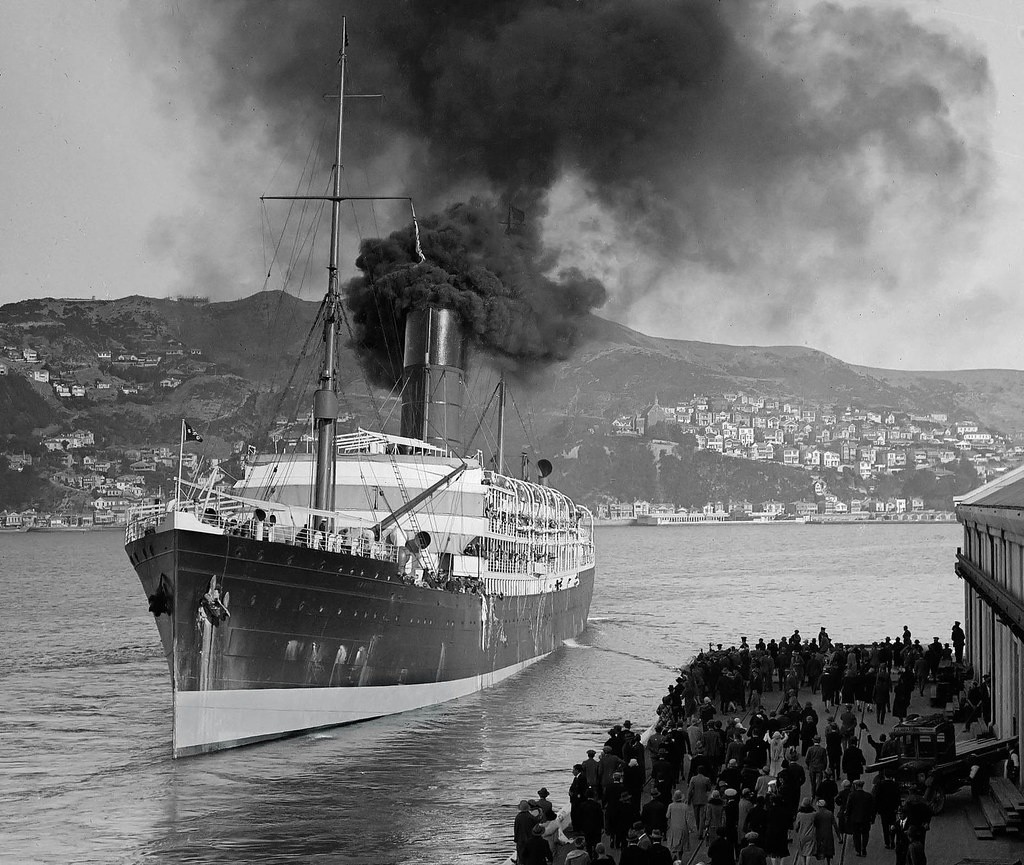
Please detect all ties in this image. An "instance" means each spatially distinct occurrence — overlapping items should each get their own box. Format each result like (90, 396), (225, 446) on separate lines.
(900, 820), (903, 830)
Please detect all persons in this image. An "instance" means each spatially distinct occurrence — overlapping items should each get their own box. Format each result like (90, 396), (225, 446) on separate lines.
(188, 496), (577, 596)
(513, 620), (992, 865)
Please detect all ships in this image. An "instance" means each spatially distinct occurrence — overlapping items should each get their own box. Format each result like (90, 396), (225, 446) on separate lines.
(125, 0), (599, 758)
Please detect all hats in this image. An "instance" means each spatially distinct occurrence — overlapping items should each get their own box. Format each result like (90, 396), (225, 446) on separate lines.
(518, 800), (531, 812)
(650, 788), (661, 797)
(816, 800), (826, 807)
(850, 736), (859, 741)
(676, 677), (683, 682)
(537, 788), (549, 797)
(944, 643), (949, 647)
(880, 663), (887, 668)
(827, 715), (834, 721)
(623, 733), (633, 739)
(532, 823), (545, 836)
(528, 799), (543, 810)
(672, 790), (685, 801)
(584, 788), (596, 798)
(668, 685), (675, 689)
(608, 729), (618, 734)
(696, 745), (705, 752)
(812, 735), (822, 742)
(722, 668), (728, 674)
(626, 828), (638, 841)
(649, 830), (663, 838)
(914, 640), (920, 643)
(845, 705), (852, 710)
(801, 798), (812, 808)
(885, 637), (891, 640)
(805, 702), (812, 707)
(587, 750), (597, 754)
(859, 721), (867, 732)
(895, 806), (905, 814)
(632, 821), (645, 830)
(623, 720), (633, 726)
(602, 746), (613, 752)
(758, 766), (770, 775)
(853, 780), (865, 785)
(745, 831), (759, 839)
(725, 788), (737, 797)
(786, 749), (800, 761)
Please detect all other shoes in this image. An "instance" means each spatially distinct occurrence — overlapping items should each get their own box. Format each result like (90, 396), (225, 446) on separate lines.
(825, 708), (830, 713)
(855, 853), (866, 857)
(884, 845), (890, 849)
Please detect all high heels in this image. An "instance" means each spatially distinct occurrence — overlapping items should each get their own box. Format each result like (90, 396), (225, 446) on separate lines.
(610, 838), (614, 849)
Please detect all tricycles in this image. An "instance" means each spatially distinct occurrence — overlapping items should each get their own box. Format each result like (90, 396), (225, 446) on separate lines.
(887, 719), (1015, 813)
